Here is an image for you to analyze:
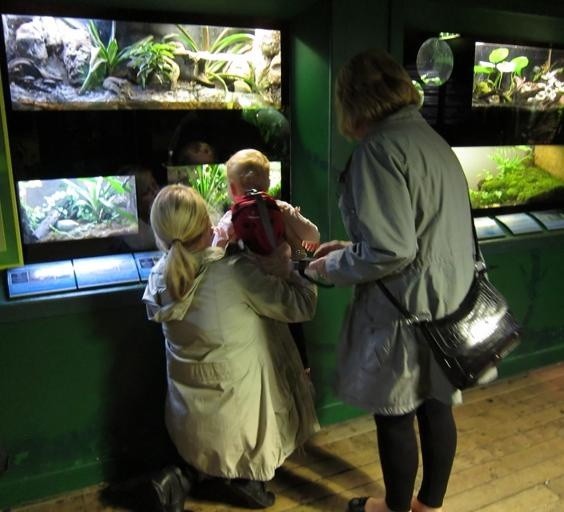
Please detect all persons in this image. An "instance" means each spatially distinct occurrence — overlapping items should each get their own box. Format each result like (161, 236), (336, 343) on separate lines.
(211, 147), (323, 374)
(284, 50), (500, 511)
(137, 182), (322, 512)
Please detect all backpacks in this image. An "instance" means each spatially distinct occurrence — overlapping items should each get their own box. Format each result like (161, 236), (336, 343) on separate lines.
(228, 188), (286, 255)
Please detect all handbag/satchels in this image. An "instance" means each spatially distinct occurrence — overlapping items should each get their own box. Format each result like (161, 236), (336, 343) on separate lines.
(420, 277), (522, 391)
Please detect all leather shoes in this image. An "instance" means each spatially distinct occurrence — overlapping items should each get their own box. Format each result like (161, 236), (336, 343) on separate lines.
(231, 476), (275, 509)
(148, 462), (191, 511)
(348, 497), (368, 512)
